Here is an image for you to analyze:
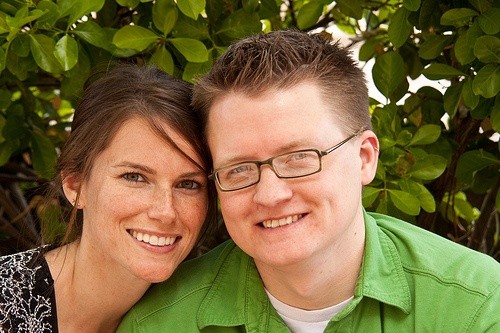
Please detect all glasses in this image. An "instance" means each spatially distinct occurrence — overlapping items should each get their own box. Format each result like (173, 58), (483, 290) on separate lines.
(208, 124), (368, 193)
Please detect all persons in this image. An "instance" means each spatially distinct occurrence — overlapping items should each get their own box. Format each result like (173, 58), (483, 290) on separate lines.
(0, 59), (217, 333)
(116, 31), (500, 333)
